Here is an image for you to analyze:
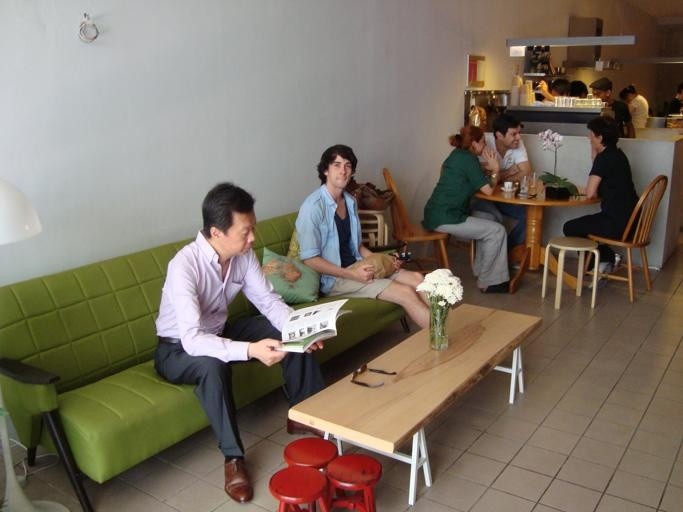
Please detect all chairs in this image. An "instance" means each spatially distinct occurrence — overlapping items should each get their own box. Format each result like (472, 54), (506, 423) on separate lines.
(583, 175), (668, 304)
(382, 167), (450, 277)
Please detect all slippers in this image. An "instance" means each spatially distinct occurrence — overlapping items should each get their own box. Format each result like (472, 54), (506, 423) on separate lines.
(483, 282), (512, 294)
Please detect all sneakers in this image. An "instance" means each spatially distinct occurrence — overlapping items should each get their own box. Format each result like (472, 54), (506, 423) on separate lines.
(588, 253), (623, 289)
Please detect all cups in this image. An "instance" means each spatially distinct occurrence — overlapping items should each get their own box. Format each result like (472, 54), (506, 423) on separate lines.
(511, 72), (535, 107)
(503, 180), (513, 192)
(552, 94), (608, 108)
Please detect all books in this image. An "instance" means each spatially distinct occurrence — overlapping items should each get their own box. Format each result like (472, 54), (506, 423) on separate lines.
(273, 299), (353, 354)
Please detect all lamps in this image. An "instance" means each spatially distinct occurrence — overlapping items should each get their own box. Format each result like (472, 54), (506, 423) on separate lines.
(0, 178), (70, 512)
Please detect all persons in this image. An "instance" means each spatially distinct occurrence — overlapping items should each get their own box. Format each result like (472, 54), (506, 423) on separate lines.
(665, 81), (683, 128)
(563, 116), (644, 291)
(294, 144), (431, 330)
(470, 113), (532, 270)
(533, 77), (649, 138)
(155, 182), (336, 504)
(421, 125), (511, 292)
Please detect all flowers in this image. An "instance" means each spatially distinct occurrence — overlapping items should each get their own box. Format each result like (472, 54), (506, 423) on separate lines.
(415, 267), (464, 348)
(536, 129), (580, 201)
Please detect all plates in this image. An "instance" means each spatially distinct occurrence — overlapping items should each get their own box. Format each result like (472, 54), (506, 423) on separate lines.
(668, 114), (683, 119)
(500, 187), (517, 194)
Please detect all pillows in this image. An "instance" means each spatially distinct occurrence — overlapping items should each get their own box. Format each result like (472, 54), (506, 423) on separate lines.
(285, 226), (303, 264)
(261, 245), (320, 304)
(344, 253), (397, 280)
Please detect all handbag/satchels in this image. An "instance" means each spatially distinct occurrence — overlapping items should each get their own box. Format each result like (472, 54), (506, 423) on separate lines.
(353, 184), (394, 210)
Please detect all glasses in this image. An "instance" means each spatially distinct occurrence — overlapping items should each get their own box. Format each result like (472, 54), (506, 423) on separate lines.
(515, 187), (537, 199)
(350, 364), (396, 386)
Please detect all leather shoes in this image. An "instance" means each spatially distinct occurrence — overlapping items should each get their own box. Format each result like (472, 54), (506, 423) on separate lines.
(224, 457), (253, 504)
(287, 419), (324, 436)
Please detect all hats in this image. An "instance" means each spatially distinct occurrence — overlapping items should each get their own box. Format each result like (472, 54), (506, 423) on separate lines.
(589, 77), (612, 92)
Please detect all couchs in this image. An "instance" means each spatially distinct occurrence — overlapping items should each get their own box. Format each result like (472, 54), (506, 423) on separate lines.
(0, 209), (410, 511)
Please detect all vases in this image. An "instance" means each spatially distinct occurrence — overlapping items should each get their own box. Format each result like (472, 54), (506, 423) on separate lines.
(544, 187), (569, 201)
(425, 296), (449, 350)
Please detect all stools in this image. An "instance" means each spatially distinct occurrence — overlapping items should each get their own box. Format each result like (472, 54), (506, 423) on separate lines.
(540, 237), (600, 310)
(325, 453), (382, 511)
(282, 437), (346, 511)
(356, 207), (386, 249)
(268, 466), (329, 511)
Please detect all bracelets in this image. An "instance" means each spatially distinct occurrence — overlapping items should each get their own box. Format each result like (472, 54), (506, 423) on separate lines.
(490, 172), (499, 179)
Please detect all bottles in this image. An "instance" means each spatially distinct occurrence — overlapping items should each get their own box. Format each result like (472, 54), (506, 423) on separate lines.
(561, 60), (565, 75)
(608, 55), (625, 72)
(520, 172), (544, 201)
(556, 64), (560, 76)
